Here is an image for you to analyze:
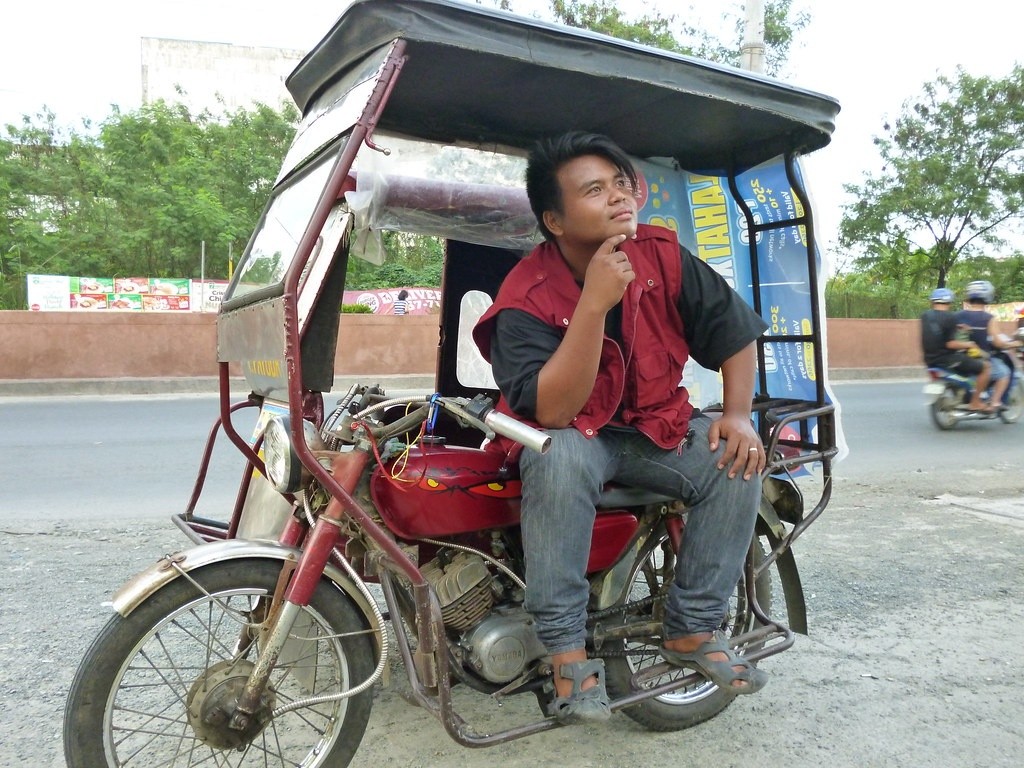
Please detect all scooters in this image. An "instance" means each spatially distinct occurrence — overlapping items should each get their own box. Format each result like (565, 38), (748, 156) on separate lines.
(925, 330), (1021, 430)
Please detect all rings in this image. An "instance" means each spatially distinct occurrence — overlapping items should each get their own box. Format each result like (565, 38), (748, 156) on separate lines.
(748, 446), (760, 453)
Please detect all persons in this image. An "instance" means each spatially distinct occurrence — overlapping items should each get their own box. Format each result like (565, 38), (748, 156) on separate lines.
(916, 287), (996, 419)
(393, 289), (410, 315)
(955, 279), (1022, 425)
(478, 125), (770, 718)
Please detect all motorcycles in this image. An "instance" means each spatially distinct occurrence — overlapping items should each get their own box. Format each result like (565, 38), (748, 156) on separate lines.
(43, 0), (837, 768)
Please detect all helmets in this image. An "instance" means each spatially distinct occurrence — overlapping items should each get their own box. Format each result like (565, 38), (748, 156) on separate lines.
(929, 287), (957, 303)
(967, 281), (995, 302)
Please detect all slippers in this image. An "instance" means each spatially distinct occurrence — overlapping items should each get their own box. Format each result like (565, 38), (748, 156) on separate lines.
(544, 656), (613, 725)
(654, 631), (768, 696)
(988, 398), (1009, 411)
(964, 404), (995, 416)
